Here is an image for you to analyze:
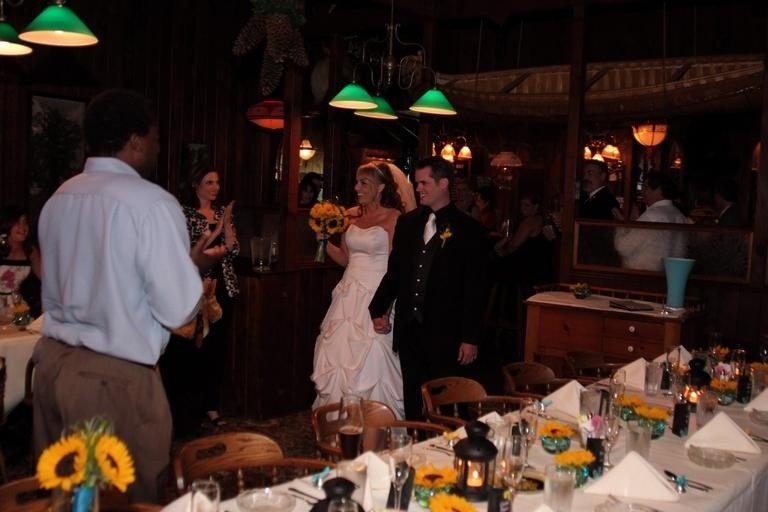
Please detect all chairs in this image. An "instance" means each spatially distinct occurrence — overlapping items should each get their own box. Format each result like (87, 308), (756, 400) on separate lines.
(499, 359), (589, 400)
(0, 476), (157, 512)
(175, 431), (334, 496)
(310, 398), (449, 455)
(420, 377), (530, 429)
(561, 347), (626, 379)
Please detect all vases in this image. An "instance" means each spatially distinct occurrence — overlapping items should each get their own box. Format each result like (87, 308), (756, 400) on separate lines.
(542, 437), (572, 455)
(640, 420), (665, 440)
(416, 486), (448, 508)
(74, 484), (96, 512)
(717, 391), (735, 406)
(618, 408), (635, 421)
(555, 466), (589, 489)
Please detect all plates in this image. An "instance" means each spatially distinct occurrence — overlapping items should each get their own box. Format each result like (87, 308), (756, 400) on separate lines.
(688, 446), (736, 469)
(234, 488), (297, 511)
(750, 409), (767, 425)
(377, 449), (427, 467)
(596, 502), (639, 512)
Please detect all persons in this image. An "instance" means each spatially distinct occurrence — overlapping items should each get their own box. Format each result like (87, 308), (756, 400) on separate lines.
(549, 208), (563, 228)
(0, 207), (40, 325)
(310, 161), (417, 452)
(475, 187), (497, 227)
(59, 134), (90, 178)
(714, 182), (741, 226)
(303, 172), (322, 194)
(614, 169), (688, 271)
(299, 179), (315, 205)
(499, 193), (547, 283)
(543, 160), (620, 266)
(171, 161), (239, 428)
(458, 184), (478, 220)
(369, 157), (497, 442)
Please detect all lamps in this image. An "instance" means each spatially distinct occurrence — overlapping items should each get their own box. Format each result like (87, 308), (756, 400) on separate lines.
(432, 127), (473, 163)
(584, 125), (623, 162)
(18, 0), (99, 47)
(680, 356), (711, 411)
(632, 113), (669, 147)
(299, 138), (320, 160)
(329, 0), (458, 121)
(0, 2), (32, 57)
(246, 99), (287, 129)
(491, 151), (523, 168)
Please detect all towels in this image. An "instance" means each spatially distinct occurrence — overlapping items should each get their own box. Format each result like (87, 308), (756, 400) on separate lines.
(685, 410), (763, 455)
(743, 387), (768, 411)
(456, 412), (501, 439)
(584, 449), (680, 504)
(653, 344), (692, 369)
(617, 357), (649, 389)
(342, 451), (391, 512)
(542, 380), (588, 420)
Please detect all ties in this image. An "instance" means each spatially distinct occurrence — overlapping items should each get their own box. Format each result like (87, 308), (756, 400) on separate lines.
(423, 213), (438, 244)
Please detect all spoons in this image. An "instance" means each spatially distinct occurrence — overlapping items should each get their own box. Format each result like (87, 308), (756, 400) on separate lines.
(663, 469), (711, 491)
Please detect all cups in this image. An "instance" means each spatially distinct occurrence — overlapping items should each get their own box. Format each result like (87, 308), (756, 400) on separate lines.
(667, 346), (680, 374)
(0, 307), (12, 328)
(602, 415), (619, 469)
(390, 434), (414, 511)
(610, 368), (626, 418)
(11, 290), (20, 308)
(545, 464), (578, 512)
(697, 392), (717, 428)
(519, 397), (538, 450)
(328, 498), (359, 511)
(644, 361), (663, 396)
(500, 437), (528, 502)
(191, 481), (219, 512)
(336, 396), (365, 461)
(626, 422), (653, 461)
(337, 463), (369, 505)
(486, 418), (511, 457)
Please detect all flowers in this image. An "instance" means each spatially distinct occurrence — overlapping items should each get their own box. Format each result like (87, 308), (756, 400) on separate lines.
(711, 380), (738, 388)
(36, 415), (134, 492)
(537, 420), (572, 437)
(415, 465), (457, 486)
(552, 448), (596, 466)
(712, 345), (730, 355)
(620, 395), (641, 405)
(308, 200), (349, 264)
(428, 491), (479, 511)
(633, 405), (668, 419)
(751, 361), (767, 377)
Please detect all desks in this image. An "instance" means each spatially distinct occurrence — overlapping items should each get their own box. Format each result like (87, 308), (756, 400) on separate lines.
(522, 291), (687, 368)
